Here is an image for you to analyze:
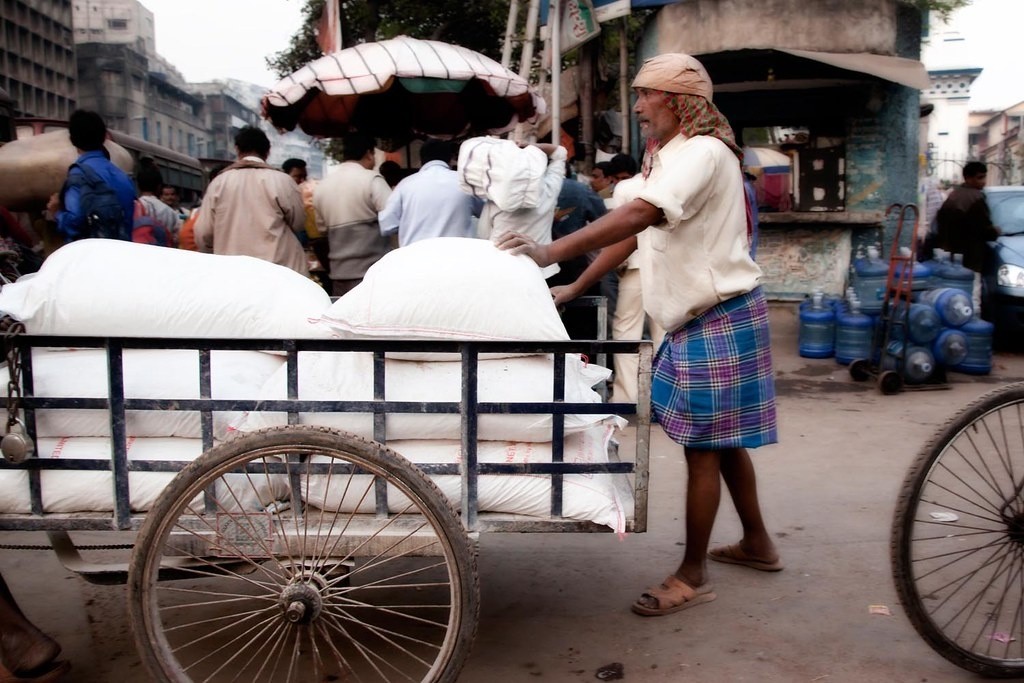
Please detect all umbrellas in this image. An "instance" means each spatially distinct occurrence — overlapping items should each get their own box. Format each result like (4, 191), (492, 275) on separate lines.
(741, 146), (791, 174)
(267, 36), (534, 171)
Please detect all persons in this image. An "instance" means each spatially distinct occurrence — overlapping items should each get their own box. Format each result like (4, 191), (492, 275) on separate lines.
(917, 221), (935, 263)
(937, 162), (1002, 271)
(0, 108), (757, 422)
(495, 51), (783, 613)
(0, 573), (72, 683)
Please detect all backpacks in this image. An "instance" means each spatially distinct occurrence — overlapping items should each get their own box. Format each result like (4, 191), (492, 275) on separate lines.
(58, 163), (130, 241)
(132, 200), (174, 248)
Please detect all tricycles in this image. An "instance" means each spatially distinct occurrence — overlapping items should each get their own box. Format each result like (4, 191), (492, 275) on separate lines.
(0, 292), (658, 683)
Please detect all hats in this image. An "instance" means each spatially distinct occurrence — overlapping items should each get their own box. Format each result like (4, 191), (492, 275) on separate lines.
(630, 52), (713, 102)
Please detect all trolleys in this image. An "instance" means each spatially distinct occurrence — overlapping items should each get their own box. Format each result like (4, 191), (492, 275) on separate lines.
(846, 201), (949, 397)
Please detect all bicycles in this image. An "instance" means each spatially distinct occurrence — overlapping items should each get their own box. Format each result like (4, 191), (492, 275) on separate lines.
(889, 379), (1024, 679)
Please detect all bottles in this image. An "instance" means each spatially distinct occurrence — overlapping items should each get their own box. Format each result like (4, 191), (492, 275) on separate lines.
(797, 244), (994, 379)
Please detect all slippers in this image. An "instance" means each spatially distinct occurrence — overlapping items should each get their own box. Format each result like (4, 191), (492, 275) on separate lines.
(632, 576), (718, 617)
(707, 542), (785, 572)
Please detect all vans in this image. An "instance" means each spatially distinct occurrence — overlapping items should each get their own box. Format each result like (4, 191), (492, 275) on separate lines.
(982, 186), (1024, 327)
